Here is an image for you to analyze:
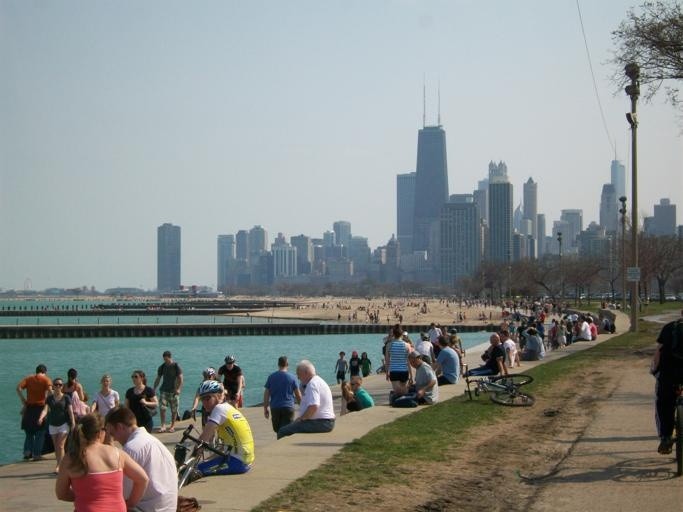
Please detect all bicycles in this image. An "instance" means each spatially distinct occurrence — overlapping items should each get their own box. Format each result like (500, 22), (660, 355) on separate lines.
(650, 369), (683, 476)
(176, 423), (232, 489)
(464, 364), (536, 407)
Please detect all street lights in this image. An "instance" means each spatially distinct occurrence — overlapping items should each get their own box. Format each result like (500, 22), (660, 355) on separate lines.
(556, 232), (564, 299)
(619, 196), (627, 313)
(623, 61), (640, 336)
(508, 265), (512, 313)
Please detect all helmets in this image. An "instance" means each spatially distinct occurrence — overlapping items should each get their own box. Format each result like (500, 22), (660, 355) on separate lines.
(197, 379), (224, 396)
(224, 354), (235, 363)
(202, 366), (215, 376)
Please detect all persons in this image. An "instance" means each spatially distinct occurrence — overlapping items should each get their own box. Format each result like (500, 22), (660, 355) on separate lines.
(218, 357), (241, 410)
(277, 361), (335, 440)
(152, 351), (184, 433)
(335, 294), (610, 415)
(192, 367), (223, 425)
(178, 380), (255, 484)
(264, 356), (301, 431)
(105, 406), (178, 512)
(90, 376), (119, 447)
(41, 386), (56, 454)
(56, 414), (149, 512)
(16, 364), (53, 461)
(238, 376), (245, 408)
(38, 378), (75, 473)
(61, 369), (85, 455)
(654, 311), (683, 454)
(650, 344), (663, 444)
(125, 370), (159, 434)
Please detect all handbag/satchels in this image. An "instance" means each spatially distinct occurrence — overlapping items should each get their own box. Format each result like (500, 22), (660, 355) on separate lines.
(143, 387), (158, 419)
(70, 382), (80, 414)
(64, 412), (80, 426)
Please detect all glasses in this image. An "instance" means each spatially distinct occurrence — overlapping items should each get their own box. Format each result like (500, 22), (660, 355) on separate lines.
(131, 375), (138, 378)
(53, 383), (63, 386)
(199, 395), (211, 402)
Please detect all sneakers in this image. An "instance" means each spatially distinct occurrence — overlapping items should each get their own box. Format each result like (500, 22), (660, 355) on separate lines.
(657, 435), (674, 454)
(167, 427), (176, 433)
(156, 426), (166, 433)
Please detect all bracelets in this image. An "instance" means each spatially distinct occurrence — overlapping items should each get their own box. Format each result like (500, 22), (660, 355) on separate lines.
(21, 399), (25, 401)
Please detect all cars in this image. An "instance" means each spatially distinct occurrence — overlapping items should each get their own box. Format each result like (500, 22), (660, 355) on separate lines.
(562, 293), (683, 302)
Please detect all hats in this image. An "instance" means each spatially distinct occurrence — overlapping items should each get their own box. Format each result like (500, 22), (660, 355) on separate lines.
(402, 330), (408, 336)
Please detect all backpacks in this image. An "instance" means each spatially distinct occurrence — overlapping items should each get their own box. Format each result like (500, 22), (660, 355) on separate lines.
(389, 391), (420, 408)
